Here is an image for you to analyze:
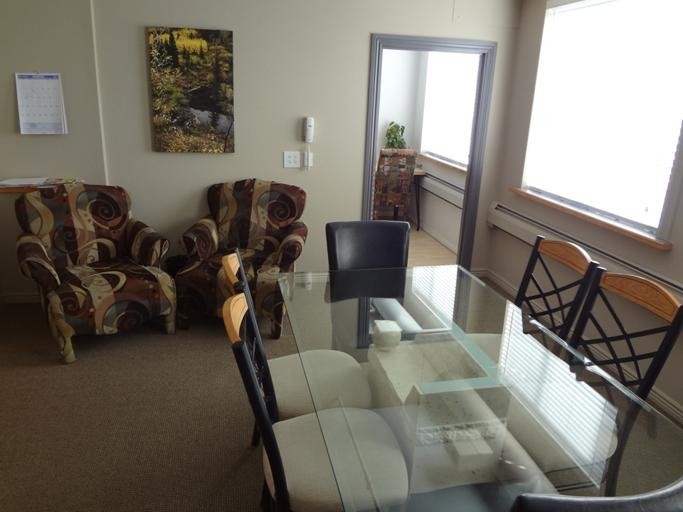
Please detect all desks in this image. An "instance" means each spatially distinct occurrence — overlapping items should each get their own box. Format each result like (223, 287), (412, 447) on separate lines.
(270, 263), (683, 512)
(375, 165), (425, 229)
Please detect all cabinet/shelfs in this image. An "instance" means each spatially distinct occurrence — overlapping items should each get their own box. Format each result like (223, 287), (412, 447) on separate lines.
(0, 178), (77, 305)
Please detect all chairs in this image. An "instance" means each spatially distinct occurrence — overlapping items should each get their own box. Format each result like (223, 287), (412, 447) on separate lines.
(499, 262), (683, 496)
(376, 148), (419, 219)
(325, 220), (410, 270)
(15, 183), (176, 364)
(432, 235), (598, 406)
(222, 281), (408, 512)
(221, 248), (373, 447)
(511, 478), (683, 512)
(175, 179), (309, 339)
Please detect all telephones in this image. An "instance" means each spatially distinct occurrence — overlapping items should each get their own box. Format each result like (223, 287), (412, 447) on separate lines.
(304, 118), (314, 144)
